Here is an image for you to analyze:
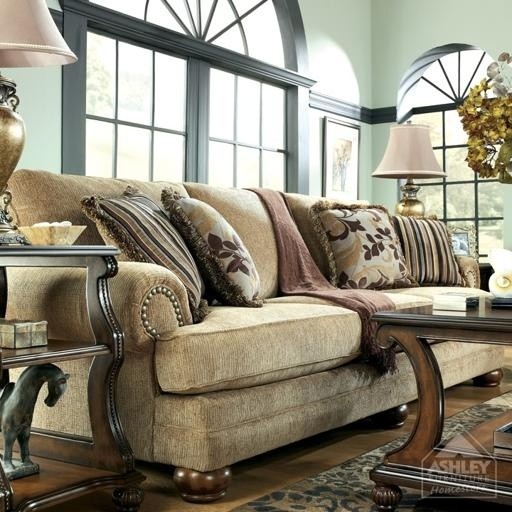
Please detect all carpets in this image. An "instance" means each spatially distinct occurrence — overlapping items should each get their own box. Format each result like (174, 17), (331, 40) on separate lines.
(227, 390), (511, 511)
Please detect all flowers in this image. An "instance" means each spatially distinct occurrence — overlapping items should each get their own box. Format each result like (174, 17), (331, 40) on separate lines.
(457, 52), (511, 186)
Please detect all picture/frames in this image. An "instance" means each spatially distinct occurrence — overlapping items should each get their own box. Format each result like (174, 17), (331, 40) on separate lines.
(322, 116), (360, 206)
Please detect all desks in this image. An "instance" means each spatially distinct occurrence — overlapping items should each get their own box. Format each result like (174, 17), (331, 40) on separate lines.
(0, 241), (146, 509)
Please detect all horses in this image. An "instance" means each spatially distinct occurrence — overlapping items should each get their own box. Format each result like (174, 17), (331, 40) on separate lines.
(0, 363), (72, 472)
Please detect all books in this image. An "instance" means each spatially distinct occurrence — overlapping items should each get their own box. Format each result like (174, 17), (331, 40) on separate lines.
(431, 309), (477, 317)
(489, 302), (512, 309)
(492, 422), (512, 450)
(487, 292), (512, 304)
(432, 292), (478, 311)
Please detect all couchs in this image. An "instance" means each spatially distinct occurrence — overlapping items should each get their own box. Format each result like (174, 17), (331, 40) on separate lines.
(9, 169), (505, 507)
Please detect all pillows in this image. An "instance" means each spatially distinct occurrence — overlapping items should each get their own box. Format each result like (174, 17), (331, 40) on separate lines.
(311, 203), (464, 290)
(83, 186), (263, 325)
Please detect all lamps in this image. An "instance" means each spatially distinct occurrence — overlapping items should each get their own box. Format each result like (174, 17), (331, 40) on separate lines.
(0, 1), (78, 247)
(373, 123), (445, 217)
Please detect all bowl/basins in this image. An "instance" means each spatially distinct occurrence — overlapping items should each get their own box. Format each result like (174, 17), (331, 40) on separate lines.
(17, 224), (89, 249)
